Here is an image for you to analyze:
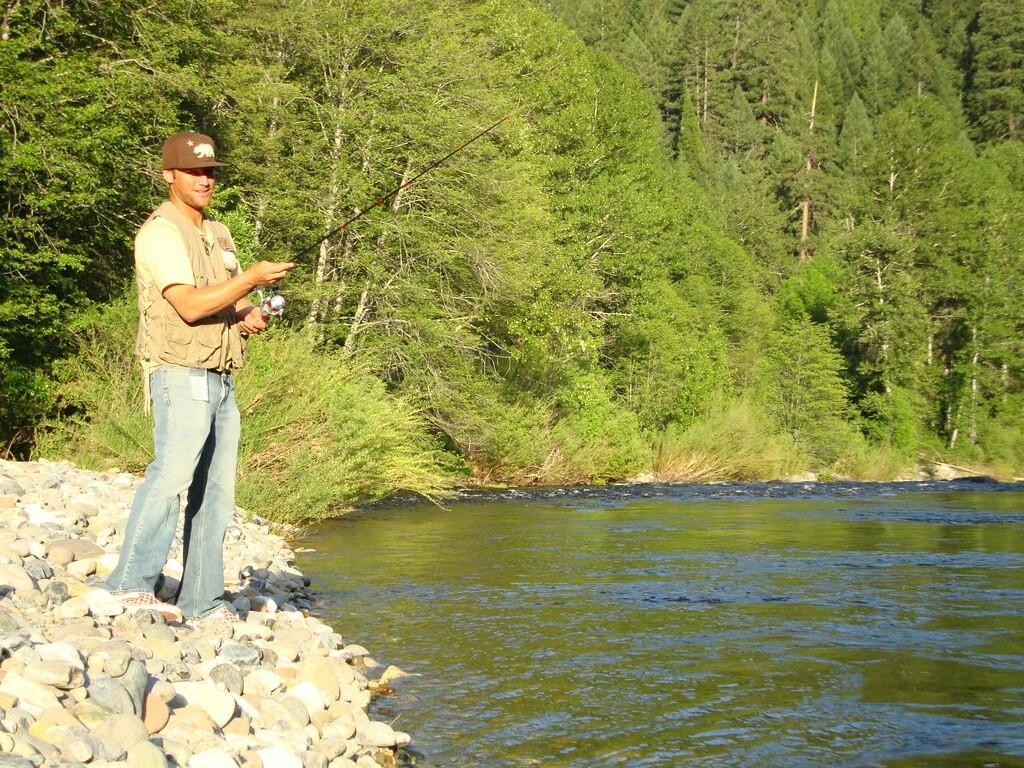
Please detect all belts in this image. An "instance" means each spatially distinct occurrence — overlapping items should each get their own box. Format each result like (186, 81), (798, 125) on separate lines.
(207, 368), (231, 375)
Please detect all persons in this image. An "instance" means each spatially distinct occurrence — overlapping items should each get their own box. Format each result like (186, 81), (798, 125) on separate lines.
(97, 133), (293, 639)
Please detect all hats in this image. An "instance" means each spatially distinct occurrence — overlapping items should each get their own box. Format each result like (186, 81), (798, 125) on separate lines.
(162, 133), (230, 169)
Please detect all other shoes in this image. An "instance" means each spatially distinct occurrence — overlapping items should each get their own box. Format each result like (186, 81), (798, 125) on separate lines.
(113, 592), (181, 621)
(182, 606), (272, 640)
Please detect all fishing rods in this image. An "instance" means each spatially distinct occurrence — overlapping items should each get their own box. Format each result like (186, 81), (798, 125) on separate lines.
(244, 114), (510, 327)
